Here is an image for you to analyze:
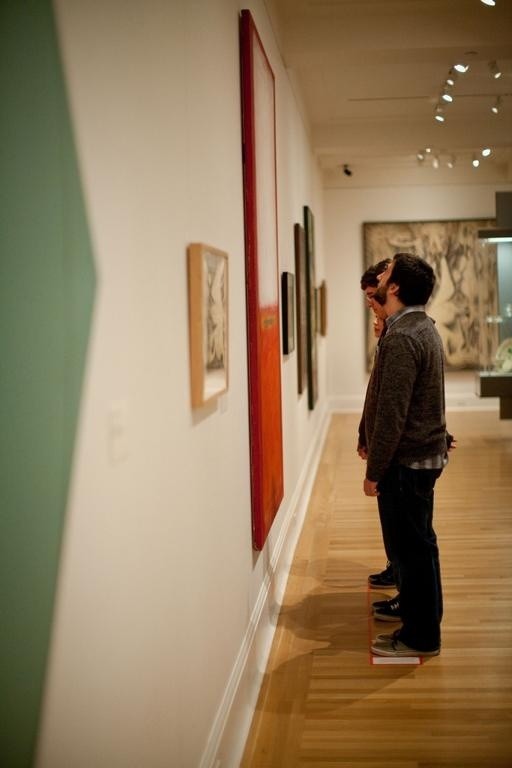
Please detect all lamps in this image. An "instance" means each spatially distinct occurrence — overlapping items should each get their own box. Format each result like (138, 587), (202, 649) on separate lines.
(480, 0), (496, 8)
(416, 59), (504, 170)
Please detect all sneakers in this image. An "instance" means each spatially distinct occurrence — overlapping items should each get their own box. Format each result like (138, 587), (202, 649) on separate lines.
(373, 601), (404, 623)
(371, 594), (400, 609)
(376, 628), (403, 643)
(367, 564), (397, 590)
(369, 639), (441, 658)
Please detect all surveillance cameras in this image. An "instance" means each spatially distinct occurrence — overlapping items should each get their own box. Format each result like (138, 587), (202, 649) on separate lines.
(343, 164), (352, 176)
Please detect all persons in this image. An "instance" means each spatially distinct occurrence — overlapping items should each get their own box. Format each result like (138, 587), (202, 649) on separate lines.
(355, 251), (457, 659)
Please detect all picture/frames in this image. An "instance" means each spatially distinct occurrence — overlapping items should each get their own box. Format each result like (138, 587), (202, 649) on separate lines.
(294, 203), (328, 411)
(188, 240), (230, 411)
(281, 271), (295, 355)
